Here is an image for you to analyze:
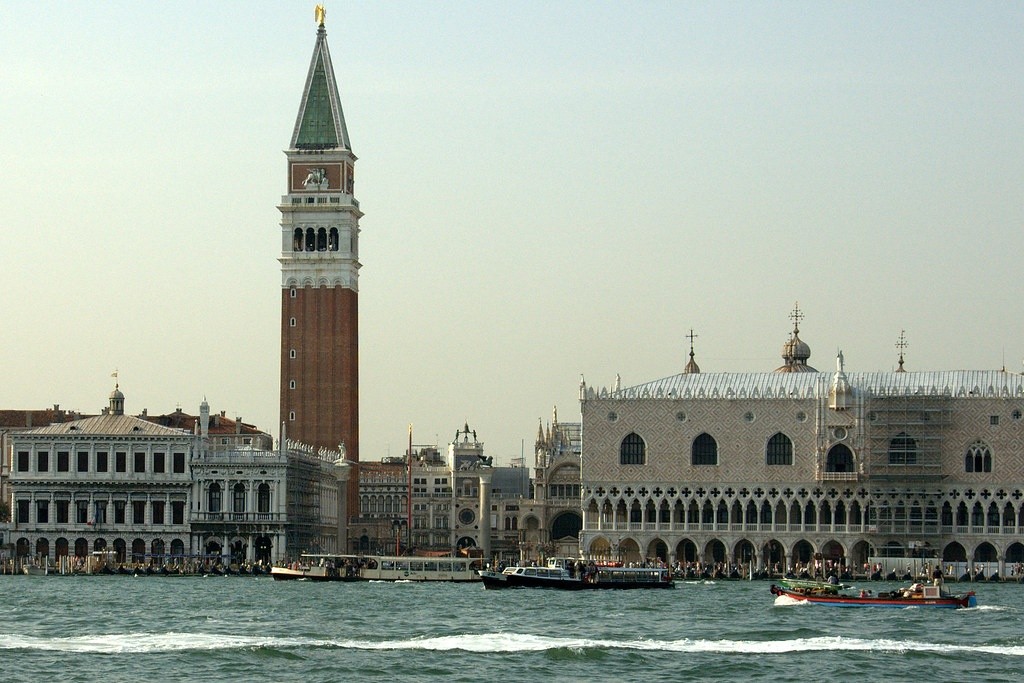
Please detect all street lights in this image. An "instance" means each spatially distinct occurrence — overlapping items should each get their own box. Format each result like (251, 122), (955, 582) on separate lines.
(617, 537), (622, 561)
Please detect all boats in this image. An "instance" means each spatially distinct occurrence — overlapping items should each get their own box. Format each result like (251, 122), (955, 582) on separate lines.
(775, 578), (852, 593)
(369, 580), (385, 584)
(395, 580), (417, 583)
(478, 556), (675, 591)
(270, 553), (491, 583)
(769, 584), (977, 610)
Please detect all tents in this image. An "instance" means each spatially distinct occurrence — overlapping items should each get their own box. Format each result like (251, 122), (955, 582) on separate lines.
(129, 553), (234, 567)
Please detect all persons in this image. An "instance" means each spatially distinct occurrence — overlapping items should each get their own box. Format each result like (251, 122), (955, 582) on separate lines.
(443, 462), (446, 467)
(473, 430), (478, 443)
(294, 239), (299, 251)
(452, 429), (459, 444)
(862, 590), (873, 597)
(486, 554), (596, 583)
(616, 373), (621, 390)
(932, 565), (944, 587)
(827, 571), (839, 584)
(328, 238), (333, 251)
(552, 405), (557, 423)
(598, 552), (1024, 578)
(274, 555), (378, 577)
(306, 243), (314, 251)
